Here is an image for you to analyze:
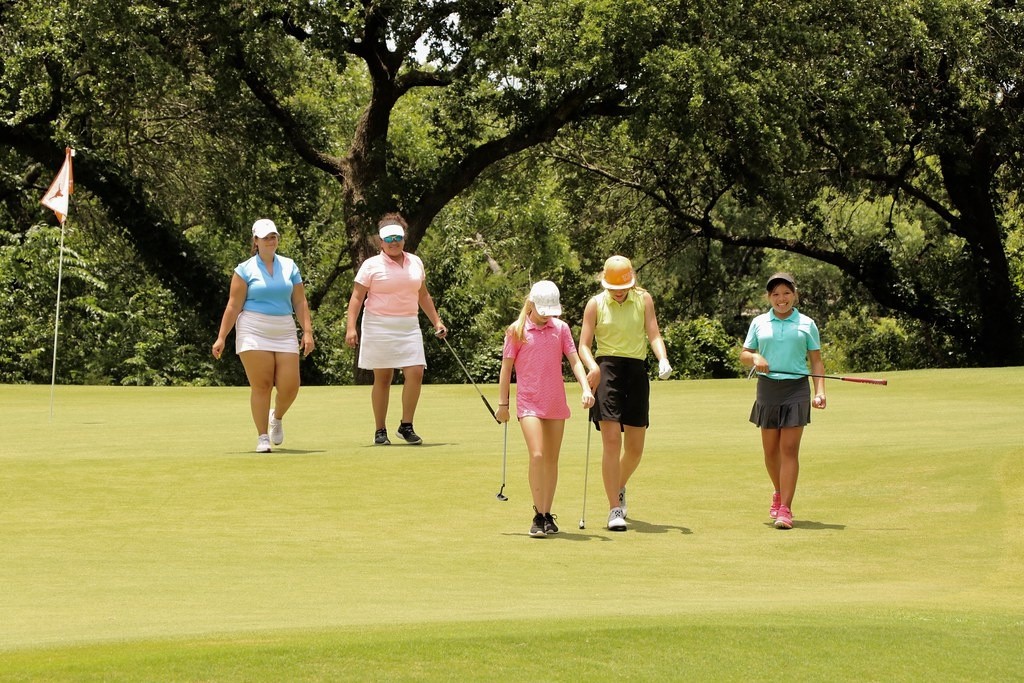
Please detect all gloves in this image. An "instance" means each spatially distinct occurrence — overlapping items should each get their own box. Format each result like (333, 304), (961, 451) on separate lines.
(659, 359), (672, 380)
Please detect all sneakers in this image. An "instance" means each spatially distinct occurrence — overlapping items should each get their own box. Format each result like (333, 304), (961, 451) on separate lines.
(256, 434), (271, 452)
(269, 409), (283, 445)
(529, 505), (559, 537)
(769, 493), (781, 519)
(373, 428), (390, 445)
(607, 486), (628, 530)
(775, 505), (793, 529)
(395, 420), (423, 445)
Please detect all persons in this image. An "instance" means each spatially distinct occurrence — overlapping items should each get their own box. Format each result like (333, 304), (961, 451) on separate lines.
(495, 280), (595, 537)
(740, 272), (826, 529)
(212, 219), (315, 453)
(345, 212), (448, 445)
(579, 255), (673, 531)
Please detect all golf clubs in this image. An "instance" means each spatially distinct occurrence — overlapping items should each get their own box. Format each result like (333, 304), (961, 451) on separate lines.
(748, 364), (888, 386)
(497, 389), (509, 502)
(434, 329), (503, 425)
(579, 407), (593, 530)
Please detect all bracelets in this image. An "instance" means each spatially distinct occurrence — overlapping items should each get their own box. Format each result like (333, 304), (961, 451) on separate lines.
(499, 404), (508, 406)
(815, 393), (824, 397)
(434, 322), (441, 328)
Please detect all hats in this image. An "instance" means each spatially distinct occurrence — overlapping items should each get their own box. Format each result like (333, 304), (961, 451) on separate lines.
(766, 272), (794, 291)
(379, 225), (404, 239)
(252, 219), (280, 238)
(529, 281), (562, 316)
(602, 256), (635, 289)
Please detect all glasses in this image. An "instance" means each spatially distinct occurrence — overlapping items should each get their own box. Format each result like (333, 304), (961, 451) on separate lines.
(384, 236), (402, 243)
